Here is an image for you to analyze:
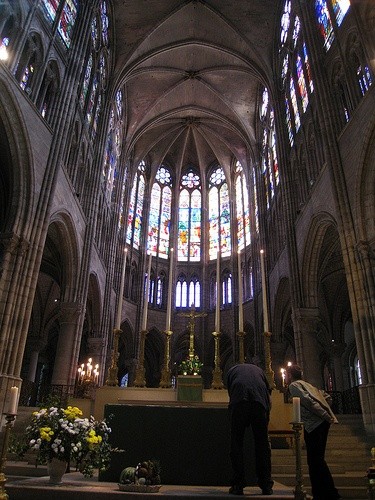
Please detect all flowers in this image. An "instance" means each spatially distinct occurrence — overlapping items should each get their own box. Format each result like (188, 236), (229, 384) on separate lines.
(8, 395), (123, 478)
(180, 356), (203, 376)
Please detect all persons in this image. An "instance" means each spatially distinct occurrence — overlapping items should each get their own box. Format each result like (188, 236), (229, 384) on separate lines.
(224, 363), (274, 495)
(287, 365), (342, 500)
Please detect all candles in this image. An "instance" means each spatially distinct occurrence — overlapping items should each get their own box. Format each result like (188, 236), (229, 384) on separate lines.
(213, 251), (222, 333)
(142, 249), (152, 331)
(112, 247), (128, 330)
(7, 385), (18, 414)
(78, 358), (99, 386)
(260, 249), (271, 332)
(236, 251), (244, 332)
(292, 395), (301, 422)
(165, 248), (174, 332)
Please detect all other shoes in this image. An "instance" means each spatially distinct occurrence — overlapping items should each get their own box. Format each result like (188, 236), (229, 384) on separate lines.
(228, 485), (244, 495)
(262, 487), (274, 495)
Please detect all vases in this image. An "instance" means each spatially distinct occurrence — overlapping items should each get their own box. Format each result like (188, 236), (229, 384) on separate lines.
(45, 457), (68, 484)
